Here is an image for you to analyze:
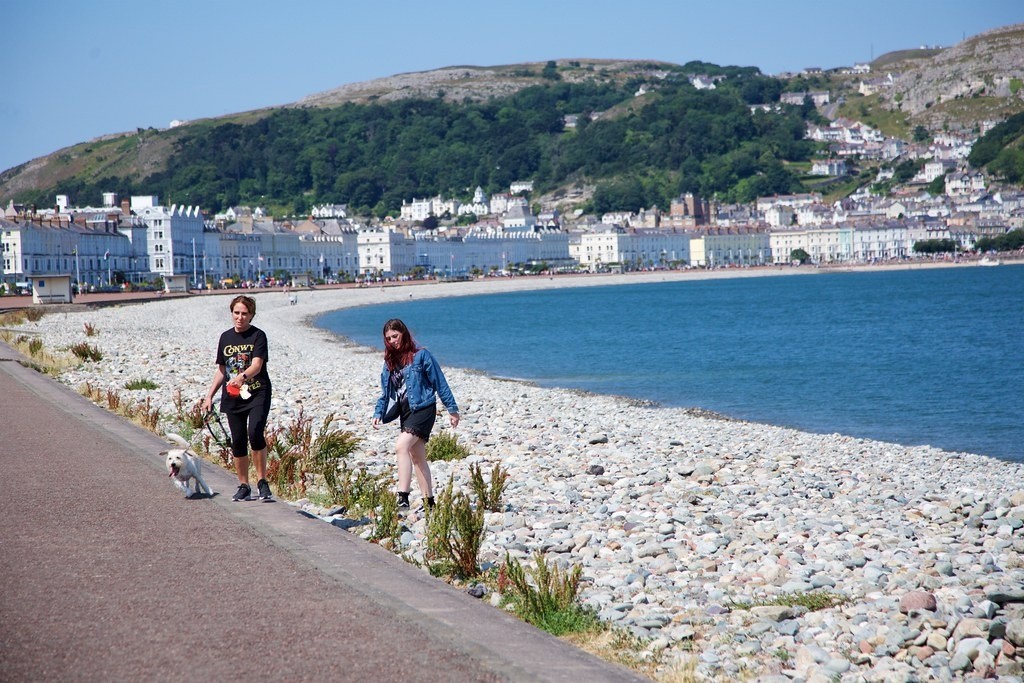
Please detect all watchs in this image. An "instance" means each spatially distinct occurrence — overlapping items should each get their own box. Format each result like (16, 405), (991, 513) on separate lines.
(241, 373), (248, 381)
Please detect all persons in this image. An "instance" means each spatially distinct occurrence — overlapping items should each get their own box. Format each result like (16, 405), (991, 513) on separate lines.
(470, 267), (558, 279)
(202, 295), (273, 502)
(71, 275), (129, 296)
(197, 276), (290, 290)
(355, 273), (415, 284)
(790, 246), (1024, 267)
(583, 260), (774, 274)
(372, 318), (460, 520)
(317, 275), (339, 284)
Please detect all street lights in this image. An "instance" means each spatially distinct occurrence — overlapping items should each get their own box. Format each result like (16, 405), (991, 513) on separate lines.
(191, 237), (196, 288)
(75, 244), (79, 293)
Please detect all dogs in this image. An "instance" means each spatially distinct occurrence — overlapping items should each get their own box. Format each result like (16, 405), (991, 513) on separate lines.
(158, 449), (214, 498)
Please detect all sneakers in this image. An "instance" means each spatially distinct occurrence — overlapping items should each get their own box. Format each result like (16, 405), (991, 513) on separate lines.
(232, 483), (251, 501)
(257, 480), (272, 499)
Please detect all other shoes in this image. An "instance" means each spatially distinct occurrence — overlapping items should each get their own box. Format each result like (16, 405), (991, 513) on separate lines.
(415, 506), (435, 513)
(397, 500), (409, 509)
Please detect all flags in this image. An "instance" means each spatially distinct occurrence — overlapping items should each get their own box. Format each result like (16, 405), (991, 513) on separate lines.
(104, 250), (110, 260)
(260, 256), (263, 261)
(72, 248), (76, 254)
